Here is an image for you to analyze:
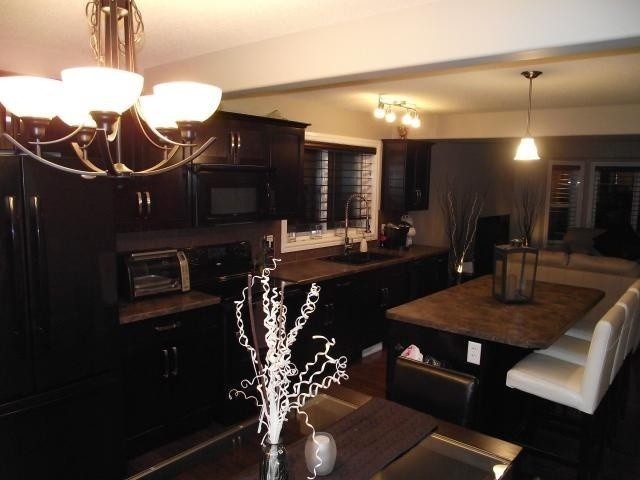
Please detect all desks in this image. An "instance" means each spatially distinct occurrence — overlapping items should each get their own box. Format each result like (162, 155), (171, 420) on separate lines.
(386, 275), (605, 426)
(125, 383), (523, 480)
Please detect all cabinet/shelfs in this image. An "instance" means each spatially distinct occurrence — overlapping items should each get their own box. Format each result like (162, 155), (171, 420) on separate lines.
(267, 118), (312, 218)
(194, 111), (269, 169)
(405, 144), (431, 211)
(120, 302), (223, 457)
(0, 105), (123, 479)
(296, 253), (449, 386)
(113, 120), (185, 233)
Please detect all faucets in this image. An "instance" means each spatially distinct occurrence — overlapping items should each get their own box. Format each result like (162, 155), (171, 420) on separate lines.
(342, 191), (372, 256)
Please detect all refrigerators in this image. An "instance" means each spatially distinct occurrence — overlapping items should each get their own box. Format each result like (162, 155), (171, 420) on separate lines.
(1, 155), (126, 478)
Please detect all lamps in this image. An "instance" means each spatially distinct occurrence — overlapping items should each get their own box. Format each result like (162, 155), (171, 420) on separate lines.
(376, 94), (421, 138)
(0, 0), (225, 183)
(514, 71), (543, 160)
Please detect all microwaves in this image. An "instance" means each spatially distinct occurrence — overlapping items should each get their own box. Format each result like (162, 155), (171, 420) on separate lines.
(191, 170), (265, 229)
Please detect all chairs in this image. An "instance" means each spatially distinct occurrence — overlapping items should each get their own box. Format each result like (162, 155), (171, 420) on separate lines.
(497, 249), (640, 480)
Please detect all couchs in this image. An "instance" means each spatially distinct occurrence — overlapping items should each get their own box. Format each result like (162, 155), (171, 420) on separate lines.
(495, 225), (636, 294)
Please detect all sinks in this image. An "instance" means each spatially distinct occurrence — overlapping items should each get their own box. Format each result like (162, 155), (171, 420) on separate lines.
(316, 249), (403, 266)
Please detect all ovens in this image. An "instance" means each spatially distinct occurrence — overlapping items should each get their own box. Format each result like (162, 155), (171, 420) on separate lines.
(119, 247), (192, 304)
